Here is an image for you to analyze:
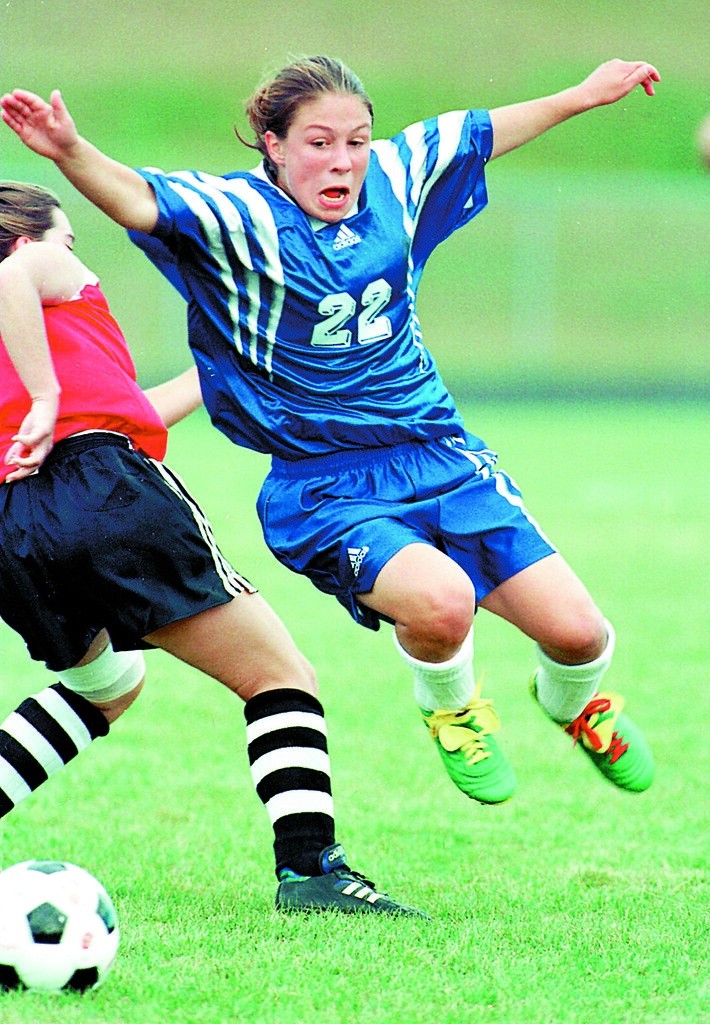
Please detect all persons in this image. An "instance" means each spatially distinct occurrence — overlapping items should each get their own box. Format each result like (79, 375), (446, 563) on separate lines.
(1, 180), (428, 917)
(1, 54), (663, 806)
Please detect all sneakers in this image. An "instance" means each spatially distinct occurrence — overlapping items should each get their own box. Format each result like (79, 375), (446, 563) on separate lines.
(276, 843), (430, 919)
(529, 666), (655, 791)
(418, 670), (517, 804)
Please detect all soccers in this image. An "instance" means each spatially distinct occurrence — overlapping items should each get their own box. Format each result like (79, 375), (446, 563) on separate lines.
(0, 860), (124, 997)
